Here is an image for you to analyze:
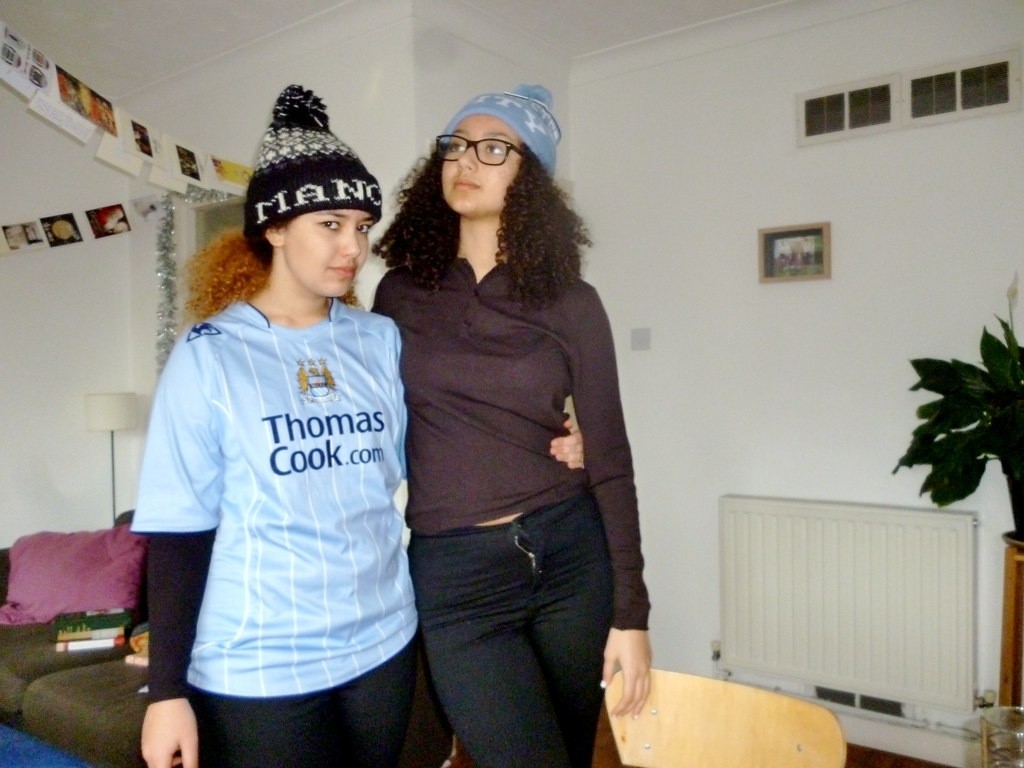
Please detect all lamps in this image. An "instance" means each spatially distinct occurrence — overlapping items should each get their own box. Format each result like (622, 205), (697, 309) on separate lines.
(85, 391), (139, 523)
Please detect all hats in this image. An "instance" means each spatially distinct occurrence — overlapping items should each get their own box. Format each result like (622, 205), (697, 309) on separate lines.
(439, 83), (562, 178)
(243, 84), (383, 237)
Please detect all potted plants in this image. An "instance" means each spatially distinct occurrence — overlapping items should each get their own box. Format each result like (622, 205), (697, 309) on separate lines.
(892, 315), (1023, 705)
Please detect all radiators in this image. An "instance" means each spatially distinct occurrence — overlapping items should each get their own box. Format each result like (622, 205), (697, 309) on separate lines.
(712, 494), (995, 716)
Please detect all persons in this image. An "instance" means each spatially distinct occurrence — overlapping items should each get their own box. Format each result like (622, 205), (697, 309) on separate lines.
(131, 83), (587, 768)
(372, 84), (652, 768)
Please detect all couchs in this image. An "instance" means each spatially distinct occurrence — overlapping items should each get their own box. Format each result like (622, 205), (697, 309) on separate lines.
(0, 510), (151, 768)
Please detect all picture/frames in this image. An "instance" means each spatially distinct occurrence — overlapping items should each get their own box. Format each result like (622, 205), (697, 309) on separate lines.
(757, 222), (831, 284)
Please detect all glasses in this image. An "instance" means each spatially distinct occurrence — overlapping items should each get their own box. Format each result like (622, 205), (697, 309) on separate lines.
(435, 134), (527, 166)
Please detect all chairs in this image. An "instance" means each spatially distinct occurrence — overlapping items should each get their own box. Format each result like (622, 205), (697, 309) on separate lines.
(605, 668), (847, 768)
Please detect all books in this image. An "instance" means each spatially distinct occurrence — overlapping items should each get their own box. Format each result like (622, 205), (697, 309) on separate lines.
(52, 605), (130, 651)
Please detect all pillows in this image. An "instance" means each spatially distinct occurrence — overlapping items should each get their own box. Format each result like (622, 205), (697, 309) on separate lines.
(0, 523), (146, 625)
